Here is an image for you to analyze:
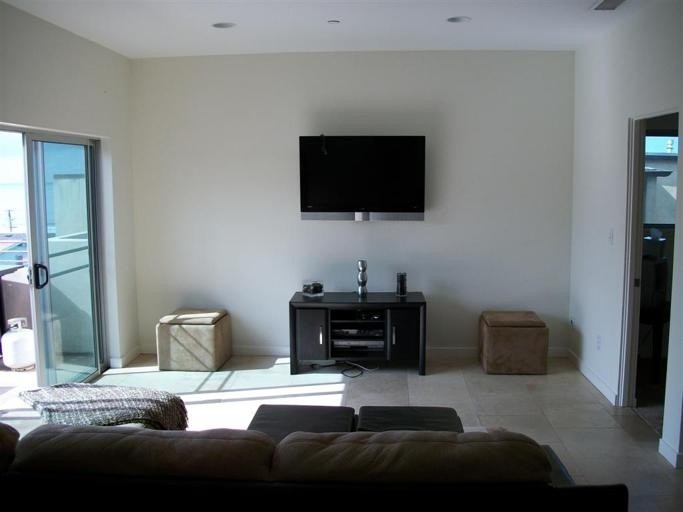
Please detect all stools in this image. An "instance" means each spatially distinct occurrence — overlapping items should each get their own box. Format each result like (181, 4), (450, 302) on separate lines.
(156, 308), (232, 372)
(356, 406), (464, 432)
(247, 404), (358, 432)
(478, 311), (549, 375)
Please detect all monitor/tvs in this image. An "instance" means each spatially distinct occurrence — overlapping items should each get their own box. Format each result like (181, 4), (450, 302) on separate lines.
(299, 134), (426, 222)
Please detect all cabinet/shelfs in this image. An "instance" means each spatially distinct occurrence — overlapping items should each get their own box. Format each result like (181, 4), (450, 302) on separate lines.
(289, 292), (426, 375)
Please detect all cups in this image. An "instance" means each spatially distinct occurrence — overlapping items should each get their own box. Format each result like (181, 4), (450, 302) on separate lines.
(396, 273), (407, 297)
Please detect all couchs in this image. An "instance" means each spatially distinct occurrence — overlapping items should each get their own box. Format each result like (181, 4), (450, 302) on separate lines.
(0, 384), (628, 512)
